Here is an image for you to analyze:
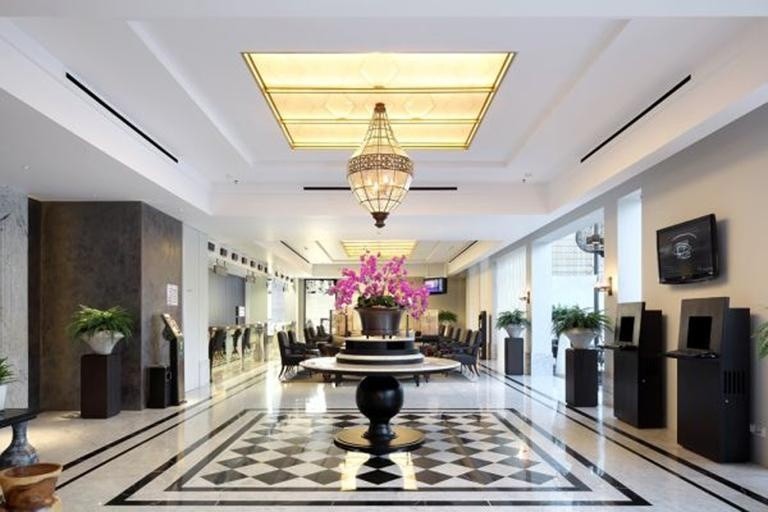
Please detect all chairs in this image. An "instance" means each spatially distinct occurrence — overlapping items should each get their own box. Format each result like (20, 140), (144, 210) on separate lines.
(289, 331), (311, 355)
(449, 330), (473, 353)
(278, 331), (304, 377)
(304, 328), (317, 343)
(448, 329), (461, 343)
(309, 327), (319, 340)
(442, 332), (484, 377)
(317, 325), (328, 337)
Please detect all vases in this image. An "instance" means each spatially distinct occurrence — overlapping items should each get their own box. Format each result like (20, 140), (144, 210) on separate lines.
(353, 304), (404, 339)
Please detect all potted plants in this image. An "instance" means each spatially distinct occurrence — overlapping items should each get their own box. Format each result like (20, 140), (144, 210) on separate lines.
(495, 310), (531, 338)
(63, 305), (137, 356)
(0, 358), (16, 410)
(437, 311), (458, 336)
(552, 306), (612, 349)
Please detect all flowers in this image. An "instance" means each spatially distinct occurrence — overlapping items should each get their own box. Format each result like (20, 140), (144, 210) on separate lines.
(326, 249), (431, 321)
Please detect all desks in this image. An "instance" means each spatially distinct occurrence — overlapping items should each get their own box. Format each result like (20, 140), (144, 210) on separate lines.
(300, 357), (461, 454)
(318, 343), (439, 383)
(0, 404), (36, 429)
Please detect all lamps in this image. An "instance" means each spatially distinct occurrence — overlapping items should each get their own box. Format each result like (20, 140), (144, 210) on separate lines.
(344, 102), (417, 230)
(240, 50), (516, 150)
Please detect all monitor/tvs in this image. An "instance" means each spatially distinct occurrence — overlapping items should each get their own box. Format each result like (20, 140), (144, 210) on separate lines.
(656, 213), (718, 284)
(423, 278), (447, 294)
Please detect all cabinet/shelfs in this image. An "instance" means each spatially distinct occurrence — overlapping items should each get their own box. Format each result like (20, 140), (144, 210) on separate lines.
(566, 349), (598, 409)
(665, 295), (754, 464)
(80, 356), (123, 417)
(612, 302), (667, 429)
(505, 338), (524, 376)
